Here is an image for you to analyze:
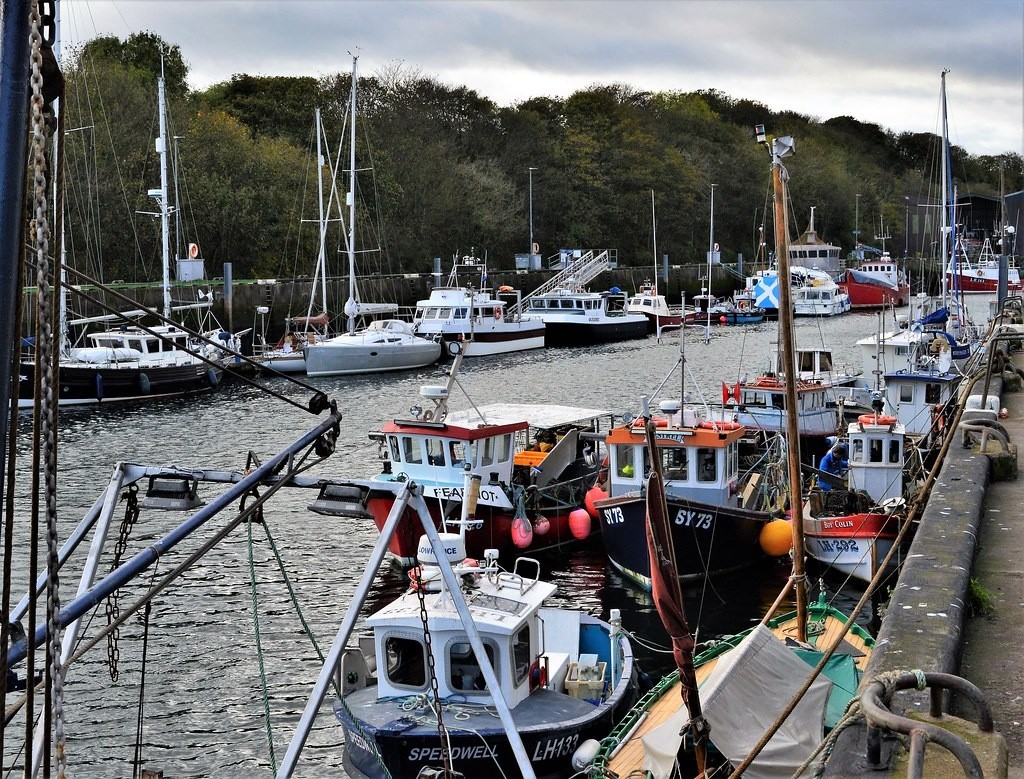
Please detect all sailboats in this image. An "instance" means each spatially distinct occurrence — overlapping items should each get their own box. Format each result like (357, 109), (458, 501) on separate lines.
(21, 41), (229, 396)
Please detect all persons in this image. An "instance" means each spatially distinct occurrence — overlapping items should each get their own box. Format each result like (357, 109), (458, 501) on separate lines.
(818, 446), (852, 491)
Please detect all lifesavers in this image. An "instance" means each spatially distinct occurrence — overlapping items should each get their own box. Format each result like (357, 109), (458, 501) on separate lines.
(756, 376), (781, 387)
(977, 270), (983, 276)
(699, 419), (741, 430)
(533, 242), (540, 253)
(931, 338), (949, 353)
(492, 305), (502, 320)
(934, 404), (945, 435)
(813, 279), (820, 286)
(189, 243), (198, 258)
(714, 243), (720, 251)
(631, 417), (669, 427)
(407, 556), (480, 591)
(857, 414), (897, 426)
(599, 455), (609, 481)
(740, 301), (748, 311)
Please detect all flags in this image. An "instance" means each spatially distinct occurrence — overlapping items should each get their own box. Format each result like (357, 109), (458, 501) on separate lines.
(733, 382), (740, 405)
(723, 382), (729, 405)
(754, 276), (781, 308)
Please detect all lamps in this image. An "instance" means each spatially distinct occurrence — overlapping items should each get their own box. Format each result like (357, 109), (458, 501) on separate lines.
(138, 474), (205, 511)
(307, 484), (374, 519)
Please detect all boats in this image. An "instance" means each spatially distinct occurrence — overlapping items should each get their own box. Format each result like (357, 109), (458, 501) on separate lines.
(250, 53), (1024, 779)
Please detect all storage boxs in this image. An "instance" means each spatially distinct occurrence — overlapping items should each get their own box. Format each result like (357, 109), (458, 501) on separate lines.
(565, 662), (607, 699)
(580, 681), (608, 706)
